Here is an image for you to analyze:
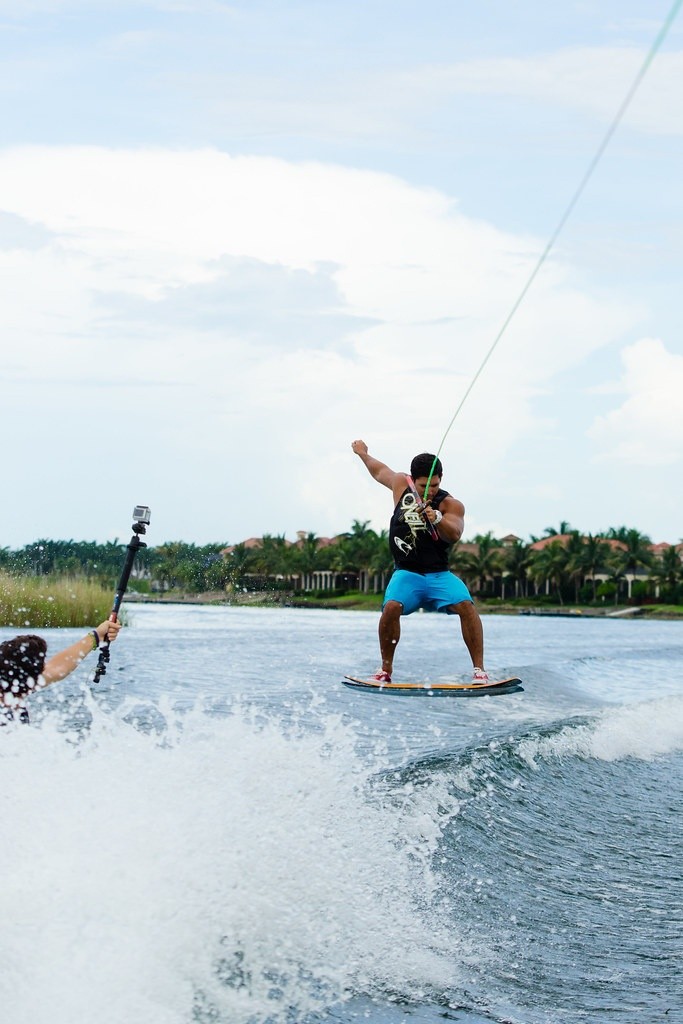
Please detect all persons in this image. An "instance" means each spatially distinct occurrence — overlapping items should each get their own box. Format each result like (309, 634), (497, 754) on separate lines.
(0, 619), (121, 726)
(351, 440), (489, 685)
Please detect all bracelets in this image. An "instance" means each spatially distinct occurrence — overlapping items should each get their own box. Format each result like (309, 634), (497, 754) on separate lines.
(88, 630), (99, 651)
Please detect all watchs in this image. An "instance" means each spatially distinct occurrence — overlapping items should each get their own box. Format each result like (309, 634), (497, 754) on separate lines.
(432, 510), (442, 525)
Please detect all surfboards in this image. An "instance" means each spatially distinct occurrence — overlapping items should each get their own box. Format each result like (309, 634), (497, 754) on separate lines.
(340, 674), (525, 697)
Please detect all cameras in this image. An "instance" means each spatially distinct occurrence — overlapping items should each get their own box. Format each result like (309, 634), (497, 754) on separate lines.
(132, 508), (146, 519)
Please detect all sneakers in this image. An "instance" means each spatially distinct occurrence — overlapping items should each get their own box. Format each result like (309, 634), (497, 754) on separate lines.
(369, 670), (392, 684)
(471, 667), (488, 684)
